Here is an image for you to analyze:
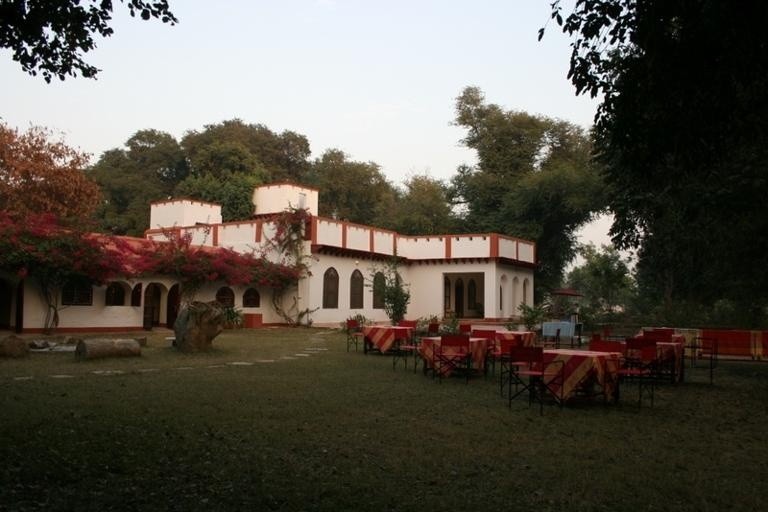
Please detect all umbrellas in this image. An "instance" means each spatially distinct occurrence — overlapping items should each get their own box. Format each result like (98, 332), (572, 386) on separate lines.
(551, 287), (584, 296)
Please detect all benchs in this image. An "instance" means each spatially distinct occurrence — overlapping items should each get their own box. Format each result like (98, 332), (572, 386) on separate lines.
(695, 327), (767, 370)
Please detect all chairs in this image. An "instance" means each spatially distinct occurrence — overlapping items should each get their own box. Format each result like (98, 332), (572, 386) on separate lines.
(497, 326), (683, 416)
(348, 320), (560, 384)
